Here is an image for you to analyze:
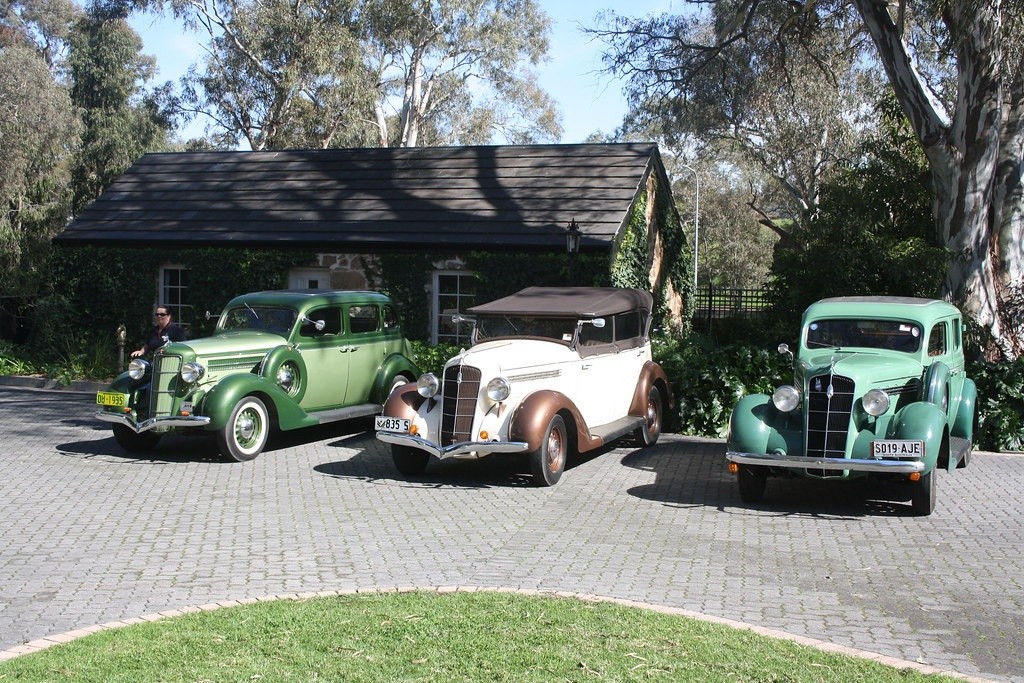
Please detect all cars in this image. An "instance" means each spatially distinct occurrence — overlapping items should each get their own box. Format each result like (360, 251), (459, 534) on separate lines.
(376, 285), (671, 488)
(725, 296), (984, 515)
(96, 290), (424, 464)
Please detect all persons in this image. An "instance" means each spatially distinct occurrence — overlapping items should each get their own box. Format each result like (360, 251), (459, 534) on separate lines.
(131, 306), (186, 357)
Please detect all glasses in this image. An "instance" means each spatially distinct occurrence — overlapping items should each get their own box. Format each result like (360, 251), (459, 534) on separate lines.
(155, 313), (169, 316)
